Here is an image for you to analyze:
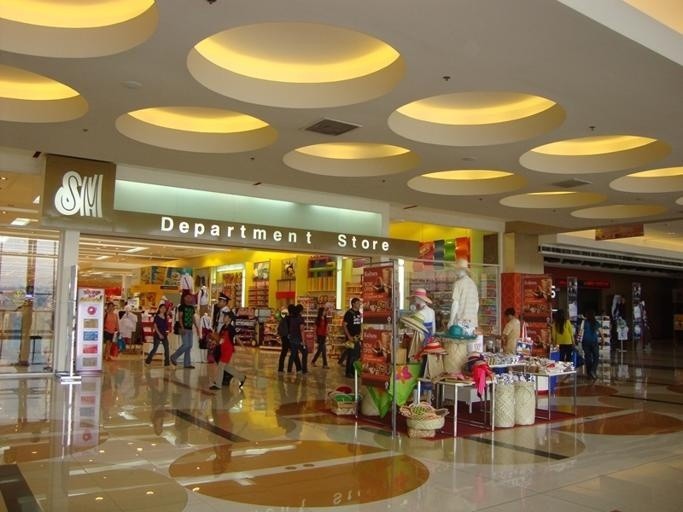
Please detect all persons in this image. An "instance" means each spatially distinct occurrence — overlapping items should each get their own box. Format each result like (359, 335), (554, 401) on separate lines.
(447, 260), (479, 333)
(277, 298), (362, 378)
(552, 311), (574, 362)
(406, 287), (436, 346)
(103, 292), (247, 390)
(501, 308), (521, 354)
(198, 286), (209, 318)
(104, 358), (361, 476)
(582, 310), (604, 380)
(181, 273), (194, 295)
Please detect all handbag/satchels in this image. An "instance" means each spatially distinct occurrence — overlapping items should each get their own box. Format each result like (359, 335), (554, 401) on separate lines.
(111, 343), (119, 357)
(576, 320), (585, 342)
(173, 321), (180, 334)
(278, 318), (288, 337)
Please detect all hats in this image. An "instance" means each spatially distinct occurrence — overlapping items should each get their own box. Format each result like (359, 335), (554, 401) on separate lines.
(407, 288), (432, 304)
(218, 293), (231, 301)
(401, 311), (476, 353)
(223, 311), (236, 319)
(464, 352), (480, 363)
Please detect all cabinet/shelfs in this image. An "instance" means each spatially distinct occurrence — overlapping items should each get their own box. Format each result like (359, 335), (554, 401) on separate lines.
(105, 283), (174, 354)
(297, 275), (558, 360)
(264, 279), (297, 347)
(234, 285), (269, 347)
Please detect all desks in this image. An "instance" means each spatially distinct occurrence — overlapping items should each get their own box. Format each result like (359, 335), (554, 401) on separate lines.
(346, 348), (580, 441)
